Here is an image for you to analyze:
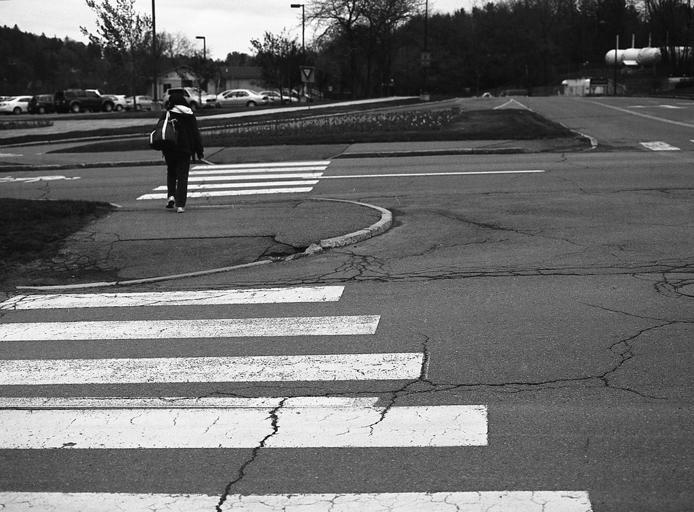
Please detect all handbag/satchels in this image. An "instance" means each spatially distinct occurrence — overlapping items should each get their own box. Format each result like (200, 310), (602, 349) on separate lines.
(149, 118), (179, 151)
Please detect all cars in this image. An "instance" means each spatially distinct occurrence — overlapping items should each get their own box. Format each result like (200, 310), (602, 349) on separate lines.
(475, 92), (493, 98)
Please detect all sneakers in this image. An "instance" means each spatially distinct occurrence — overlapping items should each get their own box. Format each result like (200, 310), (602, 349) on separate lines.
(176, 207), (185, 213)
(165, 196), (175, 209)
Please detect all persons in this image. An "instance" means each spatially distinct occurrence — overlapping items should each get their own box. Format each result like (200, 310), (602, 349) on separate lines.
(153, 94), (203, 214)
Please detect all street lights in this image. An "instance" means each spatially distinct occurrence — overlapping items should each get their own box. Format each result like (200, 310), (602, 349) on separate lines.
(195, 36), (206, 93)
(289, 3), (305, 67)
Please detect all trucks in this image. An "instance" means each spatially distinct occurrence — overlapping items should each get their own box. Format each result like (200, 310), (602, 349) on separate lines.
(497, 89), (528, 98)
